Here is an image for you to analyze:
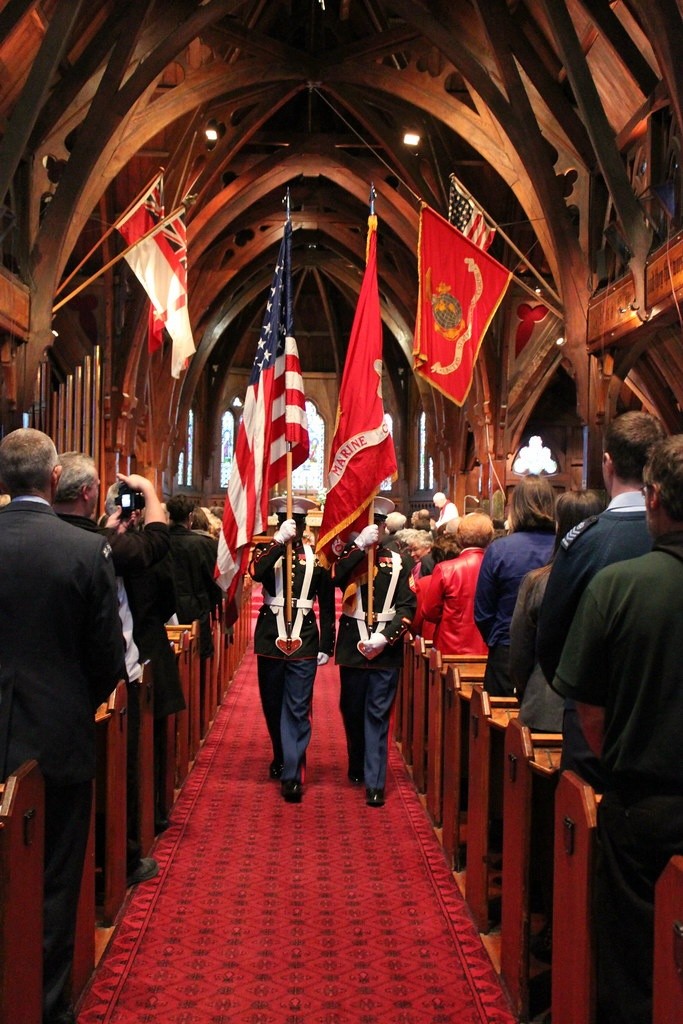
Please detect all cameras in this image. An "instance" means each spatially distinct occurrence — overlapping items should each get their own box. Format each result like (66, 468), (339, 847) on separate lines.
(114, 484), (145, 519)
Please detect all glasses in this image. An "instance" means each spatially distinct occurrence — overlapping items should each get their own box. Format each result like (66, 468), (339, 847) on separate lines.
(639, 484), (653, 496)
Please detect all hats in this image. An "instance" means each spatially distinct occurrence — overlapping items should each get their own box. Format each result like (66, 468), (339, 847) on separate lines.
(268, 496), (316, 517)
(374, 496), (395, 519)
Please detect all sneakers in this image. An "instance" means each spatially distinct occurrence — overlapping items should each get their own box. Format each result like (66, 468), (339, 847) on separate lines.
(96, 858), (159, 900)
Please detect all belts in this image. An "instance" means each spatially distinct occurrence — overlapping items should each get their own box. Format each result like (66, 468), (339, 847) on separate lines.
(343, 604), (396, 622)
(265, 597), (313, 608)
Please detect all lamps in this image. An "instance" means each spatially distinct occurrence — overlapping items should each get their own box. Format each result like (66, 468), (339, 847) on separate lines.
(206, 123), (220, 140)
(403, 126), (420, 147)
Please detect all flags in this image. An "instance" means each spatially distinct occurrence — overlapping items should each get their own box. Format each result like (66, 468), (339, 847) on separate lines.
(313, 213), (400, 614)
(412, 202), (514, 409)
(122, 207), (197, 380)
(446, 174), (499, 255)
(114, 174), (166, 355)
(211, 217), (309, 594)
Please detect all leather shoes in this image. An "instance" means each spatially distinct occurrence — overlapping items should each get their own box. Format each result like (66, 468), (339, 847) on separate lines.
(282, 779), (301, 802)
(365, 788), (385, 806)
(270, 758), (284, 778)
(348, 767), (364, 785)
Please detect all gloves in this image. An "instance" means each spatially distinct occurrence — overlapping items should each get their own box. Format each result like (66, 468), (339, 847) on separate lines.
(274, 519), (297, 545)
(363, 633), (388, 653)
(317, 652), (329, 666)
(354, 524), (379, 551)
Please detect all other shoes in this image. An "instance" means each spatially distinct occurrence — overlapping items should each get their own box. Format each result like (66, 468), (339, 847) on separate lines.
(154, 816), (169, 835)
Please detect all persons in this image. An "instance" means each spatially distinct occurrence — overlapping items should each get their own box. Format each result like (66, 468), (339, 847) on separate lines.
(0, 428), (226, 1024)
(536, 413), (670, 799)
(247, 497), (336, 803)
(335, 495), (417, 807)
(374, 474), (608, 740)
(551, 432), (683, 1024)
